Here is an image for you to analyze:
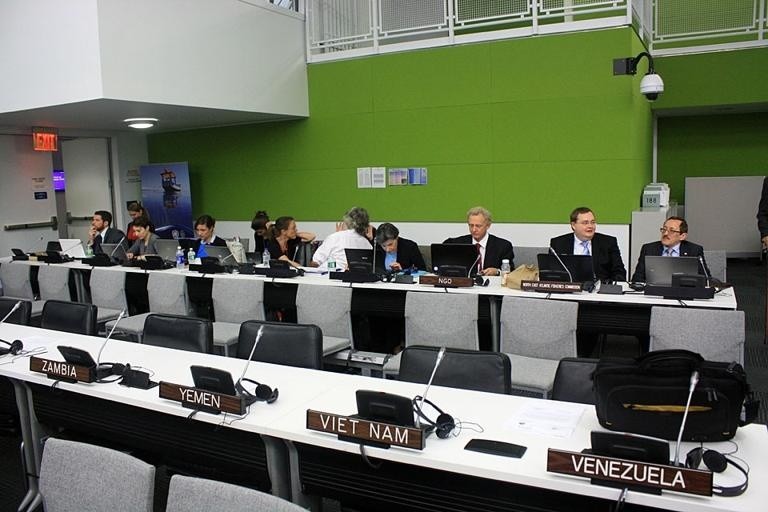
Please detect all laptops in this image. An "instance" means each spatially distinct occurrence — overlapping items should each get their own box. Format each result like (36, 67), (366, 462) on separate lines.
(11, 238), (306, 279)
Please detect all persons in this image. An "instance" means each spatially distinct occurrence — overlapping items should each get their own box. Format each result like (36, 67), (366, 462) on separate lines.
(632, 216), (711, 283)
(370, 223), (428, 274)
(262, 217), (316, 272)
(310, 206), (377, 272)
(126, 216), (162, 261)
(756, 175), (768, 247)
(250, 210), (276, 263)
(443, 206), (515, 275)
(190, 215), (227, 257)
(544, 208), (627, 284)
(85, 211), (130, 255)
(125, 202), (156, 248)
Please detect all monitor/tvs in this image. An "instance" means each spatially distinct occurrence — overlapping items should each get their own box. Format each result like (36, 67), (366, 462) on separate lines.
(431, 243), (477, 277)
(536, 253), (593, 283)
(645, 256), (698, 285)
(344, 250), (373, 271)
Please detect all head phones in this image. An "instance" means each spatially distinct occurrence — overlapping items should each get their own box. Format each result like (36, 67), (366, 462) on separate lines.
(413, 395), (455, 438)
(706, 277), (722, 293)
(381, 270), (396, 283)
(91, 362), (130, 383)
(601, 275), (618, 286)
(236, 378), (279, 403)
(471, 274), (490, 287)
(685, 447), (748, 496)
(0, 339), (23, 355)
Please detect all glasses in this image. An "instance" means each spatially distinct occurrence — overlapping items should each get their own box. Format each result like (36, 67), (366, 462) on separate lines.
(659, 228), (681, 235)
(574, 221), (597, 226)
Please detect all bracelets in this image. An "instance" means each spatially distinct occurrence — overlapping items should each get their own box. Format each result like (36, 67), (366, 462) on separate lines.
(496, 268), (499, 277)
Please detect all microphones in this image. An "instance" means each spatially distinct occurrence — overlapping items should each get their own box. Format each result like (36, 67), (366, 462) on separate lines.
(549, 246), (573, 283)
(674, 370), (700, 465)
(414, 345), (447, 430)
(468, 255), (482, 277)
(235, 324), (264, 390)
(96, 306), (126, 368)
(372, 237), (377, 273)
(698, 256), (710, 287)
(0, 301), (22, 325)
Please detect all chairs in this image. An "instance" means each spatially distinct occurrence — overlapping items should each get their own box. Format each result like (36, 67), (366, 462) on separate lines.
(1, 237), (768, 511)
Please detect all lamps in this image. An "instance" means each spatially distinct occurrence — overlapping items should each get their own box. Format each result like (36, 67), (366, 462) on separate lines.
(124, 117), (159, 129)
(612, 50), (663, 104)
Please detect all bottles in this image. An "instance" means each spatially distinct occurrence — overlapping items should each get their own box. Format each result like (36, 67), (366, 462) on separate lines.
(174, 245), (195, 267)
(261, 249), (270, 267)
(500, 259), (510, 286)
(327, 257), (336, 273)
(84, 243), (93, 258)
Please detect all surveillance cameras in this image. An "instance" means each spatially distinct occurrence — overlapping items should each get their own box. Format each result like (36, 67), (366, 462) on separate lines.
(640, 73), (664, 100)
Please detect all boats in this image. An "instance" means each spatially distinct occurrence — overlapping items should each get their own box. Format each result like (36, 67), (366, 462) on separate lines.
(160, 169), (181, 192)
(163, 188), (180, 209)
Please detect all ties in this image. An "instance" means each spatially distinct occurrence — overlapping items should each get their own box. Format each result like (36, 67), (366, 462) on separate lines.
(475, 243), (483, 274)
(581, 241), (590, 255)
(666, 249), (674, 257)
